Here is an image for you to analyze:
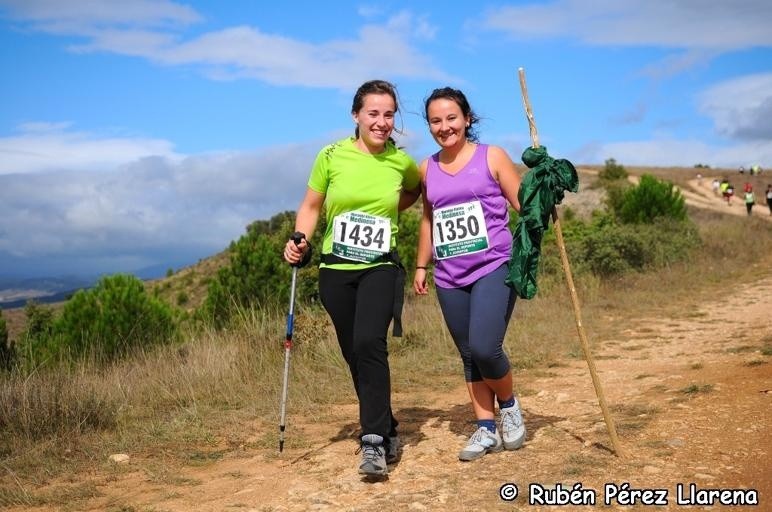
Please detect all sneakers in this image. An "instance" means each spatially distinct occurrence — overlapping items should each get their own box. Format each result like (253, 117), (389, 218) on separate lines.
(383, 429), (401, 465)
(459, 427), (503, 461)
(359, 434), (387, 475)
(500, 397), (525, 449)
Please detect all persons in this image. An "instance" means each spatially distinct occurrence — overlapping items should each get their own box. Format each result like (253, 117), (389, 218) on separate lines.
(414, 87), (526, 461)
(697, 164), (772, 216)
(283, 81), (421, 476)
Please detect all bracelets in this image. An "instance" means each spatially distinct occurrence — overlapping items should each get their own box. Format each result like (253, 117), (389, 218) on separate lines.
(416, 267), (429, 269)
(306, 239), (312, 252)
